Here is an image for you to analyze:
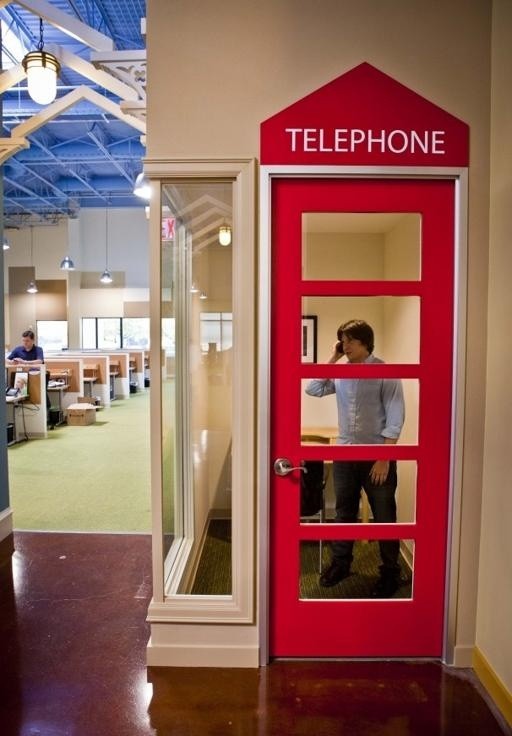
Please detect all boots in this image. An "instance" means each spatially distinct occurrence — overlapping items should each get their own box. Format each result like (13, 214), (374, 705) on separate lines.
(318, 554), (354, 587)
(369, 565), (402, 599)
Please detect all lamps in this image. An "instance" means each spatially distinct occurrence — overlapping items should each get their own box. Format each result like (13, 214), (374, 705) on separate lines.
(59, 172), (76, 271)
(99, 191), (113, 283)
(132, 172), (152, 200)
(21, 15), (59, 106)
(2, 237), (9, 250)
(218, 218), (232, 247)
(189, 280), (200, 293)
(27, 225), (39, 294)
(200, 291), (207, 299)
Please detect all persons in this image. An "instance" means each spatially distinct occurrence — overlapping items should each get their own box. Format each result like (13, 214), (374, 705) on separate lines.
(6, 330), (44, 364)
(305, 320), (404, 598)
(16, 378), (27, 395)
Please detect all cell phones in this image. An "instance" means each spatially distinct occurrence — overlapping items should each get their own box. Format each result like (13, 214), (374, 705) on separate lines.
(336, 342), (344, 353)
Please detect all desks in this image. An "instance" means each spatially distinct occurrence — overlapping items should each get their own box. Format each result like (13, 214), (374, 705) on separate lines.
(302, 443), (371, 544)
(5, 357), (150, 447)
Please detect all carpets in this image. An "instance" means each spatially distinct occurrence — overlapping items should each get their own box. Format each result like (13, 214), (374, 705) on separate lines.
(8, 375), (177, 534)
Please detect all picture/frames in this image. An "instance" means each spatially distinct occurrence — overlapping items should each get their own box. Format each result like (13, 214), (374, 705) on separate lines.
(300, 314), (317, 365)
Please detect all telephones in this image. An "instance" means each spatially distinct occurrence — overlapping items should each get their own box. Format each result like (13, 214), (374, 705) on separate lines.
(5, 386), (19, 396)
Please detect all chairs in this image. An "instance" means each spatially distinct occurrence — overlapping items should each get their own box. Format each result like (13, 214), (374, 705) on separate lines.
(300, 459), (326, 575)
(301, 434), (331, 447)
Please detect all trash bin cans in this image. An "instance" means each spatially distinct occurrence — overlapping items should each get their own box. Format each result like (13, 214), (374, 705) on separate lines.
(145, 377), (150, 386)
(130, 382), (138, 393)
(92, 396), (101, 411)
